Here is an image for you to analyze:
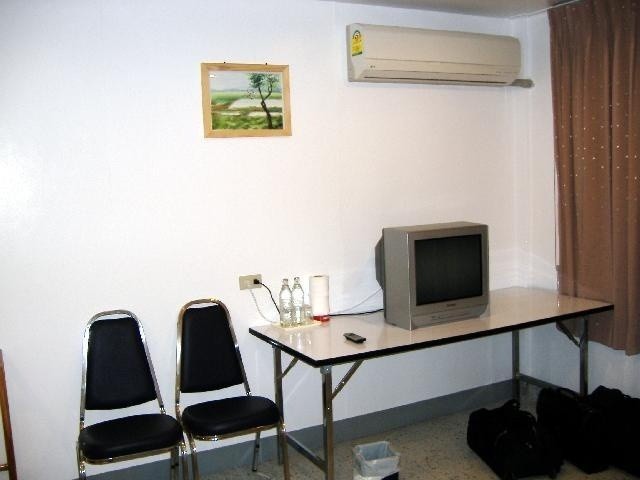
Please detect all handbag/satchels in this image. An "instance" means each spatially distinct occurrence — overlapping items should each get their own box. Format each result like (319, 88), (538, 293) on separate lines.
(464, 397), (556, 480)
(584, 384), (640, 479)
(535, 380), (613, 475)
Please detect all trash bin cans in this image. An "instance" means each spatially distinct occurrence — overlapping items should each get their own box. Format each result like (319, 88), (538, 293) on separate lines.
(354, 441), (399, 480)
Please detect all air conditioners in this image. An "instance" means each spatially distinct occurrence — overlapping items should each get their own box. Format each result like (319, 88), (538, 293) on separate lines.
(346, 24), (535, 88)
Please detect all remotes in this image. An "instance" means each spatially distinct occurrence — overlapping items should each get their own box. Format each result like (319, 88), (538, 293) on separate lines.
(344, 332), (366, 344)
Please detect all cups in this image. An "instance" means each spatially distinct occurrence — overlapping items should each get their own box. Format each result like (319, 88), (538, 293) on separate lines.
(302, 305), (314, 326)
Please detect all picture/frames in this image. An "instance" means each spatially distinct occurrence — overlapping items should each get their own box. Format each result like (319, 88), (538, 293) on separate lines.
(200, 62), (294, 137)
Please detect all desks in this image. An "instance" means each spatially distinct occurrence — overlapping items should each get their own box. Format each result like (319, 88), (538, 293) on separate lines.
(248, 285), (614, 480)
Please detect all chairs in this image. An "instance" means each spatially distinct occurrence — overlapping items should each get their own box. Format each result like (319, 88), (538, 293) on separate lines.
(174, 299), (291, 479)
(76, 310), (190, 479)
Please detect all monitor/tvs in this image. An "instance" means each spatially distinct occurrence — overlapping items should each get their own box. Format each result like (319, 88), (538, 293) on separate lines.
(375, 221), (489, 331)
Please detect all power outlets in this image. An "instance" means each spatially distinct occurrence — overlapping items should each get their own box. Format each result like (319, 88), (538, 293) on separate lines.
(238, 273), (263, 290)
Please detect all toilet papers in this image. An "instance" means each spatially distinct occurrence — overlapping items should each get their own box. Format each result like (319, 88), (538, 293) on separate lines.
(308, 274), (330, 316)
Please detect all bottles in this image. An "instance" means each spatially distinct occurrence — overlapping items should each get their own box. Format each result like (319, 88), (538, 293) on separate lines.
(279, 276), (304, 328)
